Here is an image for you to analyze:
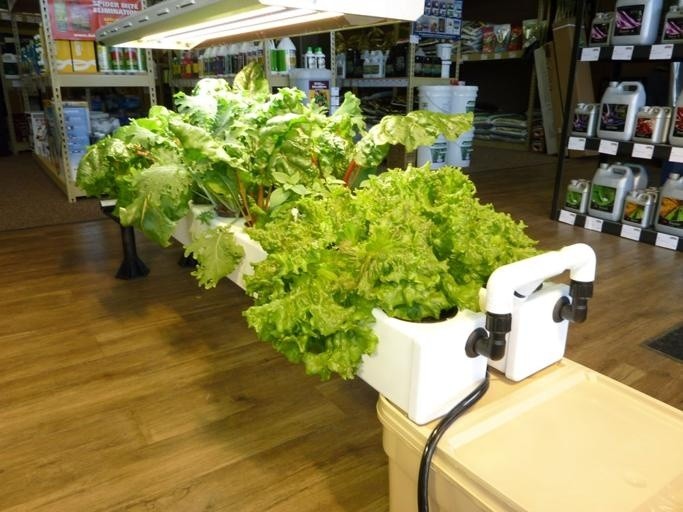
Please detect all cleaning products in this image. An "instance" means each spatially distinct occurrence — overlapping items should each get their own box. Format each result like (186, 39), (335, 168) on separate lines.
(305, 47), (325, 68)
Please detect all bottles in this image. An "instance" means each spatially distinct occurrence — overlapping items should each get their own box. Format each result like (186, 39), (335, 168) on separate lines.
(173, 55), (198, 79)
(346, 48), (360, 79)
(304, 47), (325, 69)
(386, 43), (407, 78)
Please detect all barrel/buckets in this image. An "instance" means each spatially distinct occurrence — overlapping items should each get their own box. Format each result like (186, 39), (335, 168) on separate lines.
(364, 50), (386, 77)
(566, 0), (683, 236)
(173, 38), (297, 79)
(336, 54), (345, 79)
(416, 86), (479, 169)
(433, 43), (452, 78)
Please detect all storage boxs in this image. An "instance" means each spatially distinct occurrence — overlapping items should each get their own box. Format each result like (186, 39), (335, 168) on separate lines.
(377, 357), (683, 512)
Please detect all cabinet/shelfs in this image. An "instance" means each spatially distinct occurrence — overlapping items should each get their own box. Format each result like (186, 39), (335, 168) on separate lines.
(0, 0), (682, 250)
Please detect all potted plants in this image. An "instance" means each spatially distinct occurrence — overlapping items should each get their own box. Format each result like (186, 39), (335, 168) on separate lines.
(75, 66), (576, 427)
